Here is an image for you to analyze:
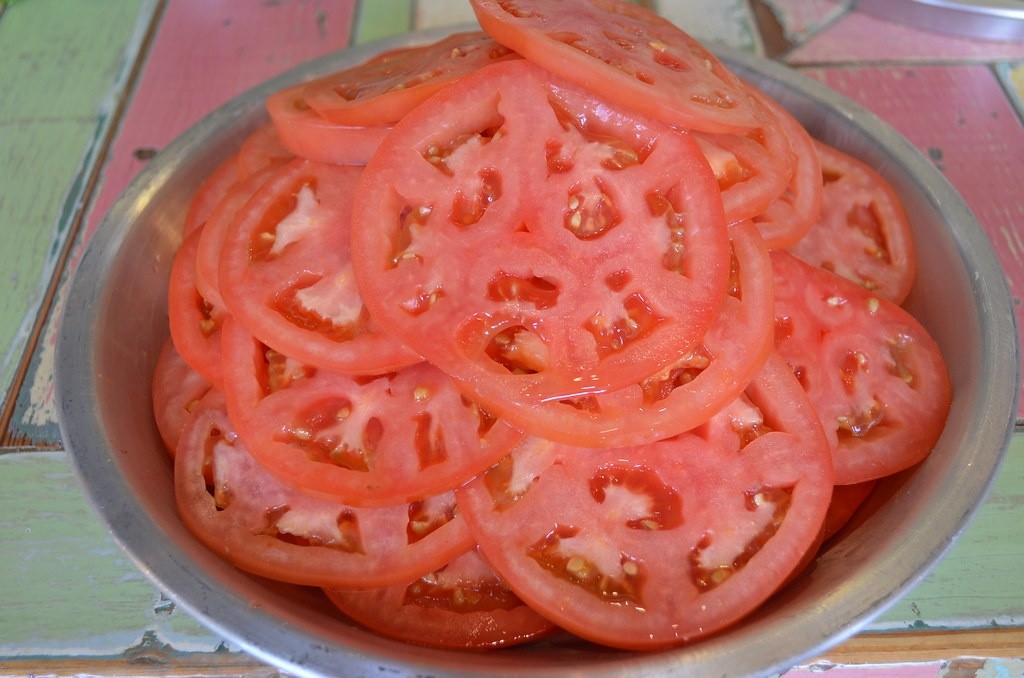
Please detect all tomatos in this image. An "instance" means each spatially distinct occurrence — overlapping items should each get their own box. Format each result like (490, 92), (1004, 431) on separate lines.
(149, 0), (954, 651)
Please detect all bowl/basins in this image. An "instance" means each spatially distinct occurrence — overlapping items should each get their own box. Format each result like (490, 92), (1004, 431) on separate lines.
(54, 21), (1019, 676)
